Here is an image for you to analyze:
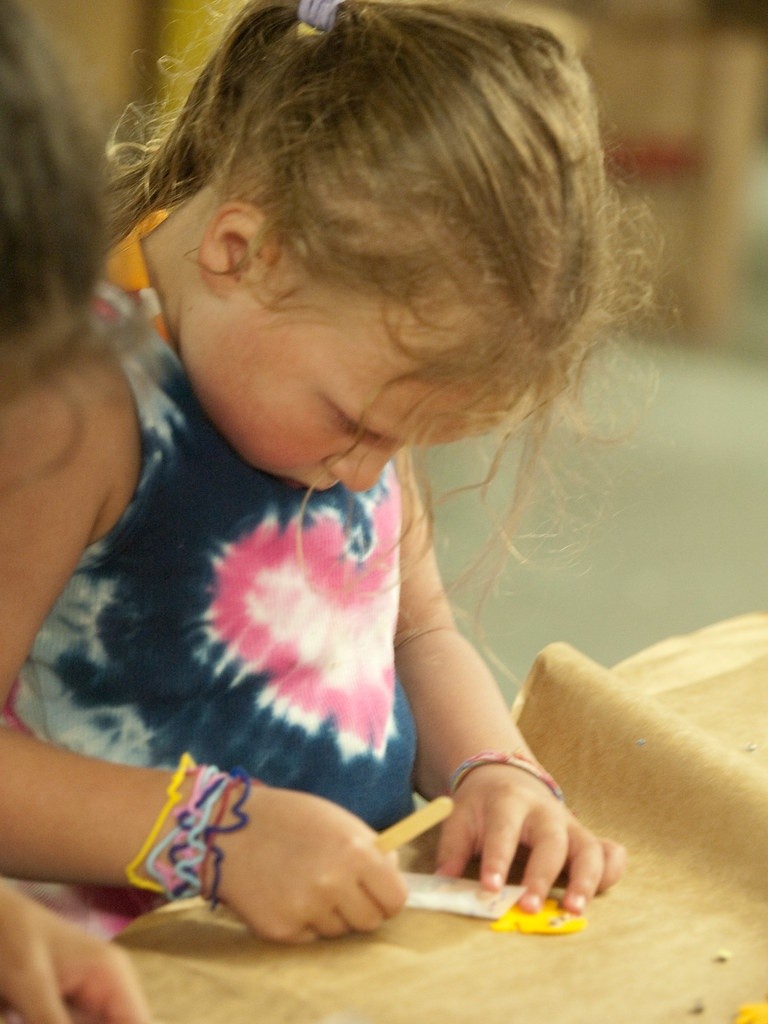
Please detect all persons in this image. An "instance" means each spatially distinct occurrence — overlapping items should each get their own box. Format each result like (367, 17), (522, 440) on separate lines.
(0, 0), (627, 947)
(0, 0), (150, 1024)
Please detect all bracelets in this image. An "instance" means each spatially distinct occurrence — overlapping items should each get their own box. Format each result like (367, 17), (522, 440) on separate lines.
(442, 751), (564, 803)
(124, 752), (252, 912)
